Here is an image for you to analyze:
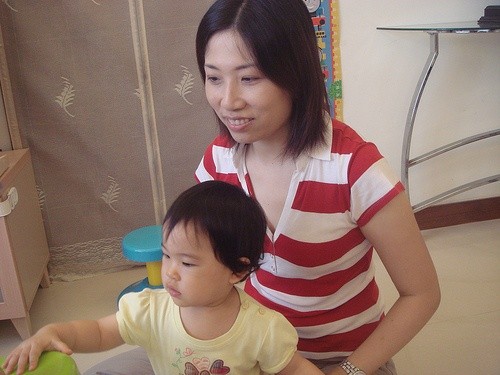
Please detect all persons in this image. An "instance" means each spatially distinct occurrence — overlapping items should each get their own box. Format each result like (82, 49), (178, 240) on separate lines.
(0, 180), (326, 375)
(83, 0), (442, 375)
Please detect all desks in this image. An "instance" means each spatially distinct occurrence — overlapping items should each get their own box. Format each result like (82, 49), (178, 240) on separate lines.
(376, 21), (500, 214)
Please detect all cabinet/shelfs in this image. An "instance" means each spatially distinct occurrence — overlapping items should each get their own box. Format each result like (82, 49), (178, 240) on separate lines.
(0, 148), (51, 341)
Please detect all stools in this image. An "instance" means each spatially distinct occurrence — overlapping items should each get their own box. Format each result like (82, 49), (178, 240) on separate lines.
(119, 225), (165, 311)
(0, 351), (78, 375)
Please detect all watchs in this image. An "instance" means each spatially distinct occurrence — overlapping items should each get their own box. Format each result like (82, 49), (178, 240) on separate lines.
(336, 358), (367, 375)
